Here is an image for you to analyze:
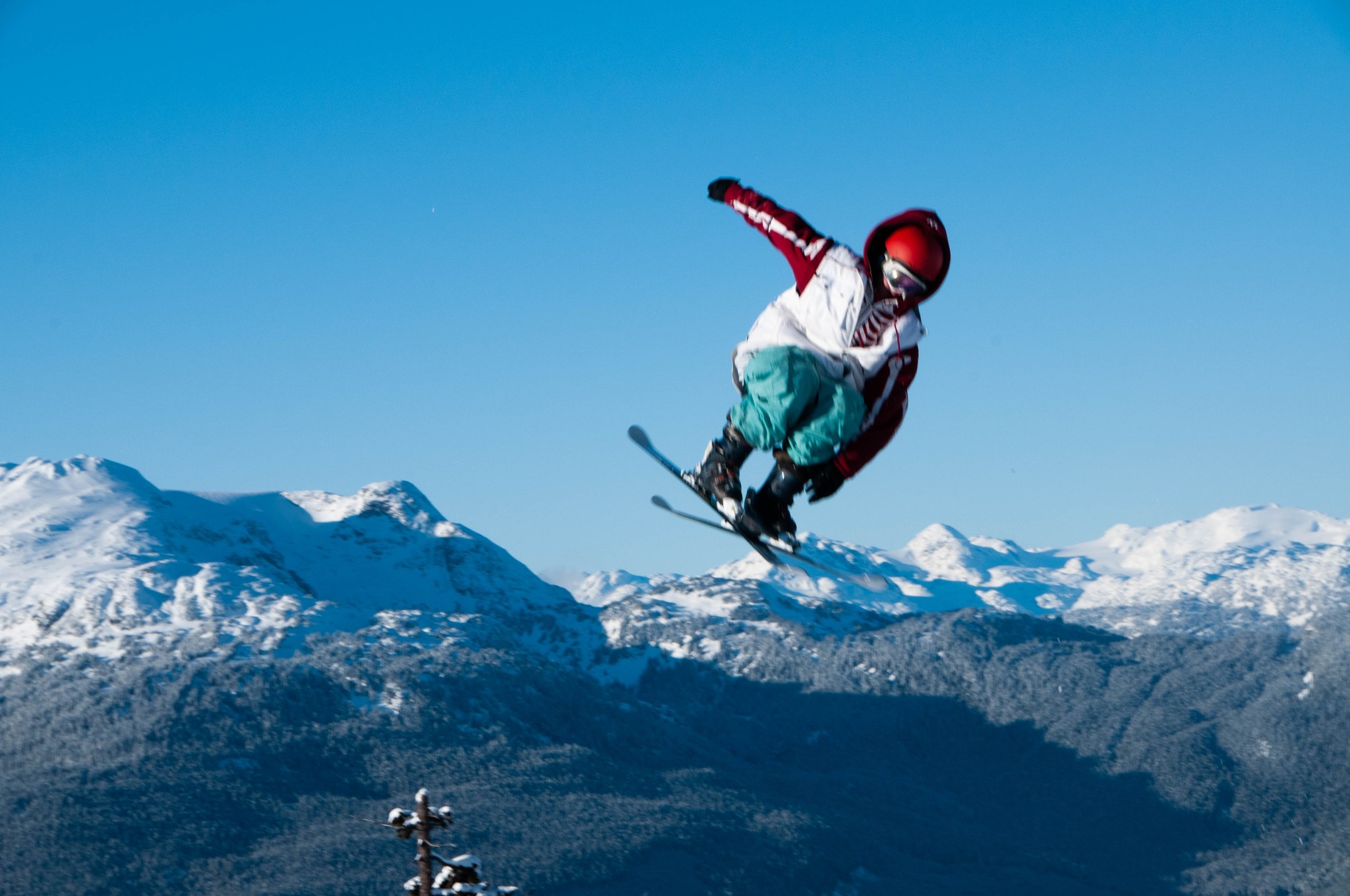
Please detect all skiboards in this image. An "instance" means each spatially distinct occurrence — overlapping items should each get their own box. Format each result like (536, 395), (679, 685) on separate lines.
(626, 424), (894, 595)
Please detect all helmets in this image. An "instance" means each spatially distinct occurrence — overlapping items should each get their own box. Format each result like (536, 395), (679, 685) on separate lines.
(884, 225), (943, 286)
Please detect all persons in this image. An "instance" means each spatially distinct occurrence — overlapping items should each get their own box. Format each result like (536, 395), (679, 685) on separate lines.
(694, 178), (950, 539)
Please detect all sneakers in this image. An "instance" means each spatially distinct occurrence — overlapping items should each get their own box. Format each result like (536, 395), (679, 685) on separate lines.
(750, 488), (797, 533)
(696, 441), (742, 501)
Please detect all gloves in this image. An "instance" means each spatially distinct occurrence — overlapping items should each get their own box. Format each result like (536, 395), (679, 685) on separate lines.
(806, 464), (844, 504)
(707, 177), (739, 202)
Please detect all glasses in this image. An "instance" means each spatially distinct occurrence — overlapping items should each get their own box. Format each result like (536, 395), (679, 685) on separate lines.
(883, 261), (925, 303)
